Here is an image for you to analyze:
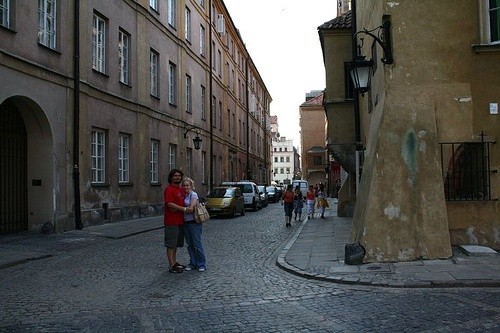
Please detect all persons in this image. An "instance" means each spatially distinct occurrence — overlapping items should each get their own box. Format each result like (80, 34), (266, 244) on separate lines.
(317, 198), (329, 219)
(318, 183), (325, 198)
(335, 179), (341, 199)
(181, 176), (207, 272)
(306, 185), (315, 220)
(164, 169), (193, 272)
(314, 184), (318, 197)
(294, 185), (303, 222)
(281, 184), (295, 227)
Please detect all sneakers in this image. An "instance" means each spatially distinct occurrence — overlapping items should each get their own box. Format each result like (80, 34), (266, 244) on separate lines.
(169, 262), (187, 274)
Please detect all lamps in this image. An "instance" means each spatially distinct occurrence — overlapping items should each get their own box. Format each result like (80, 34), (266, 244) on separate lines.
(184, 126), (202, 150)
(348, 14), (393, 97)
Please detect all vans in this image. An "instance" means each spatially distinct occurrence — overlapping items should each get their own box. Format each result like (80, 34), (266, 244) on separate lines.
(237, 182), (259, 211)
(292, 180), (308, 199)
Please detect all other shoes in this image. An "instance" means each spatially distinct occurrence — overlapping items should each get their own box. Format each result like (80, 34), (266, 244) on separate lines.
(286, 223), (291, 228)
(198, 268), (205, 271)
(185, 267), (192, 271)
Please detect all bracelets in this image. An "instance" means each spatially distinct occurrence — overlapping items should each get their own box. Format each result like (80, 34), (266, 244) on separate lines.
(184, 207), (186, 212)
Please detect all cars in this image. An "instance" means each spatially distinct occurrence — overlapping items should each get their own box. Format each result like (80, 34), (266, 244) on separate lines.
(201, 182), (245, 219)
(256, 186), (284, 208)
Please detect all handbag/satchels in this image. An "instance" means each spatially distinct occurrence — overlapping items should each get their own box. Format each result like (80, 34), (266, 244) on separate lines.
(190, 192), (210, 224)
(298, 200), (303, 208)
